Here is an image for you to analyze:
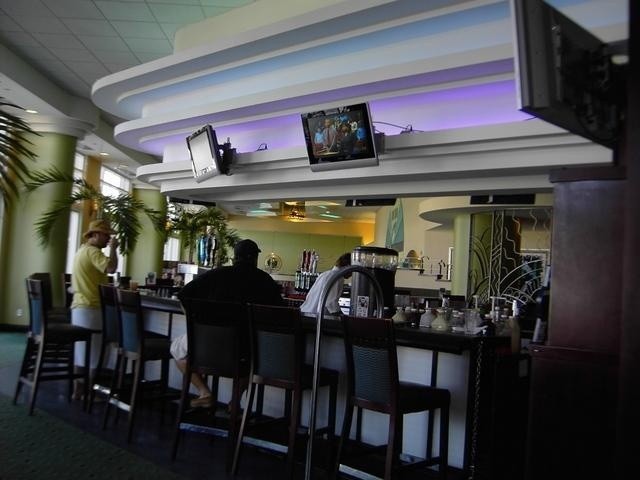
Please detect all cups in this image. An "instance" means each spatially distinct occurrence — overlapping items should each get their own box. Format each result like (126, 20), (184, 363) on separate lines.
(107, 238), (119, 247)
(464, 309), (476, 334)
(129, 280), (138, 291)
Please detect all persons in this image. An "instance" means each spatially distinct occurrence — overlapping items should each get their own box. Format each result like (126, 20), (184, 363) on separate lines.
(300, 252), (354, 314)
(168, 239), (283, 414)
(314, 117), (365, 158)
(71, 219), (120, 402)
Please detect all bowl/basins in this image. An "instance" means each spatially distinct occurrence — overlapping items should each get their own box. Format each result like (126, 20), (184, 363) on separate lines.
(489, 297), (506, 311)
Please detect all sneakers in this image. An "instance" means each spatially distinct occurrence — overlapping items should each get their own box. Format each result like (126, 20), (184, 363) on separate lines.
(189, 393), (216, 409)
(69, 391), (107, 404)
(226, 399), (241, 415)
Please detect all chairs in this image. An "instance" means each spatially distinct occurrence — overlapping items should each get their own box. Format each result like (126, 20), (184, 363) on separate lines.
(101, 287), (173, 447)
(13, 269), (102, 418)
(333, 314), (451, 480)
(85, 284), (142, 430)
(169, 296), (262, 474)
(227, 302), (339, 479)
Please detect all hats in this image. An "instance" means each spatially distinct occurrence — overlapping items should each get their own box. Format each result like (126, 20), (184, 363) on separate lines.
(233, 238), (262, 258)
(82, 219), (117, 241)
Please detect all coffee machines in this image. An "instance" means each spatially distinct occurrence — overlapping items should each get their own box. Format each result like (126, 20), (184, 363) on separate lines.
(346, 243), (399, 321)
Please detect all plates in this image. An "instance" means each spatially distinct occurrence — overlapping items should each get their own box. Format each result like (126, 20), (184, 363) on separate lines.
(450, 326), (467, 332)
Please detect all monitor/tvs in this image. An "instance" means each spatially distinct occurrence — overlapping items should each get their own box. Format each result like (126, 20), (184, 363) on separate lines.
(186, 123), (223, 183)
(510, 0), (614, 148)
(301, 103), (379, 172)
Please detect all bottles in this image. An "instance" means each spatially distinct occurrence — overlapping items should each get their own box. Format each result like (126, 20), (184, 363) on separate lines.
(419, 308), (434, 327)
(512, 300), (518, 311)
(491, 306), (501, 322)
(383, 305), (425, 326)
(429, 309), (448, 332)
